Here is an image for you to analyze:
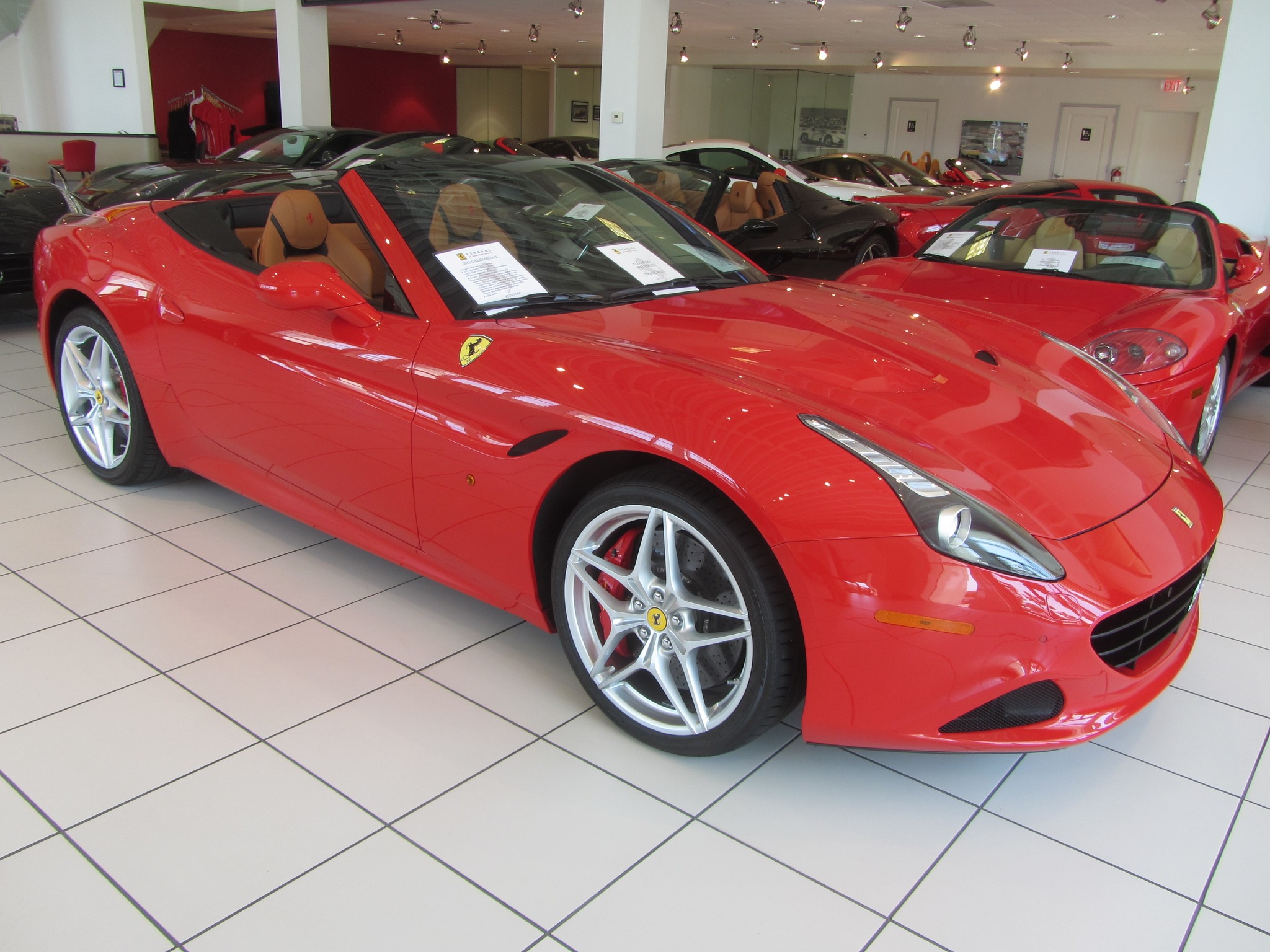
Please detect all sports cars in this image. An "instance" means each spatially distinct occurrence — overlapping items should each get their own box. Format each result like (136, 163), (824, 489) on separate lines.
(25, 153), (1227, 754)
(0, 123), (1270, 468)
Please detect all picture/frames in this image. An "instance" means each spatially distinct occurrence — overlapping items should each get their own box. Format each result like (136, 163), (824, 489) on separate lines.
(112, 68), (126, 88)
(571, 101), (589, 123)
(593, 106), (600, 120)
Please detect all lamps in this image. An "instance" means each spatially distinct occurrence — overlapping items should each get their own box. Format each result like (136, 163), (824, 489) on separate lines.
(441, 50), (452, 63)
(1183, 77), (1198, 95)
(1202, 0), (1224, 29)
(1061, 53), (1074, 70)
(476, 39), (487, 55)
(678, 47), (689, 64)
(550, 48), (559, 63)
(393, 30), (404, 46)
(568, 0), (584, 19)
(527, 23), (543, 42)
(894, 7), (915, 33)
(751, 29), (766, 47)
(429, 10), (443, 31)
(669, 12), (684, 36)
(1014, 41), (1029, 62)
(816, 42), (829, 61)
(962, 25), (978, 50)
(873, 53), (884, 70)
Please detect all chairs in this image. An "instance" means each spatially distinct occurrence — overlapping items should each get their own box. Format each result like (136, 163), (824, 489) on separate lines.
(651, 173), (687, 211)
(717, 179), (761, 230)
(929, 158), (950, 181)
(1013, 216), (1084, 269)
(430, 182), (521, 270)
(48, 140), (102, 191)
(252, 186), (380, 302)
(915, 158), (927, 174)
(848, 162), (866, 181)
(1146, 227), (1201, 284)
(824, 161), (841, 178)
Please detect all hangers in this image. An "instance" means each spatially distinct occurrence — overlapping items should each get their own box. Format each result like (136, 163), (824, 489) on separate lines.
(167, 91), (191, 111)
(203, 87), (223, 111)
